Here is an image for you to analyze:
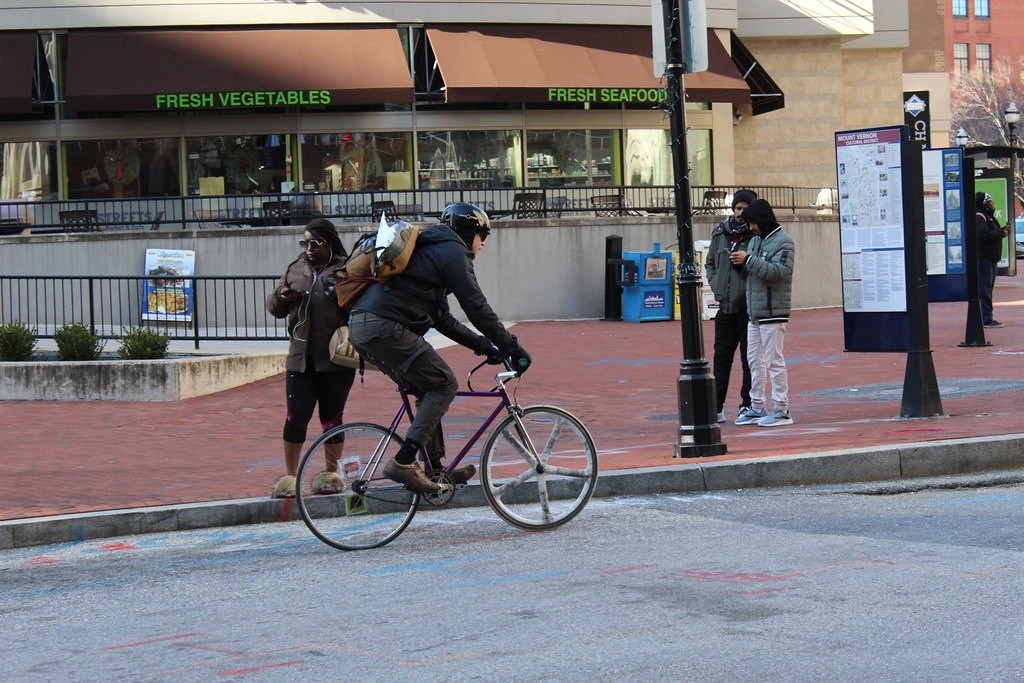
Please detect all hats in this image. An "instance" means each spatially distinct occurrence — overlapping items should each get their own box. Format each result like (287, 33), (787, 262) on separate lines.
(732, 189), (757, 211)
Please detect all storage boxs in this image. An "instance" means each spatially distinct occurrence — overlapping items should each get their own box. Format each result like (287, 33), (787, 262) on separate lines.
(623, 241), (719, 323)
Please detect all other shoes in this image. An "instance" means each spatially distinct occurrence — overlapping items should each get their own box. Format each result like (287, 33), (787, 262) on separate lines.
(311, 471), (345, 494)
(271, 475), (304, 498)
(738, 407), (749, 415)
(718, 410), (726, 422)
(983, 319), (1003, 328)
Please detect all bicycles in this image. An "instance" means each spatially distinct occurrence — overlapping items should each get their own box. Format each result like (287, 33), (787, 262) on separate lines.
(294, 347), (599, 552)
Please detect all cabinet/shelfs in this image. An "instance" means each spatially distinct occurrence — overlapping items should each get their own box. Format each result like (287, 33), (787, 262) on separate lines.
(417, 167), (514, 182)
(528, 161), (610, 181)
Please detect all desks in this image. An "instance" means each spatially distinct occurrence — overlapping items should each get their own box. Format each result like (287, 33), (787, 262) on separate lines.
(219, 218), (291, 226)
(0, 223), (32, 235)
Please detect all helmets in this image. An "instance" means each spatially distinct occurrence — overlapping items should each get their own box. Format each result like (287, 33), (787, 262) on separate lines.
(440, 201), (491, 250)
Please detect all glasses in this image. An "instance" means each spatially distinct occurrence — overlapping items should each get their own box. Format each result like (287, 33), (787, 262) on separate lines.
(299, 239), (326, 248)
(476, 229), (487, 242)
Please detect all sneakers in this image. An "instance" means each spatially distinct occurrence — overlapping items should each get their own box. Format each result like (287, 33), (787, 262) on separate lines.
(757, 408), (793, 427)
(734, 407), (771, 425)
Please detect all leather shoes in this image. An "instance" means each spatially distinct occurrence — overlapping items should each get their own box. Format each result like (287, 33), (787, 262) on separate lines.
(381, 456), (441, 493)
(431, 464), (477, 498)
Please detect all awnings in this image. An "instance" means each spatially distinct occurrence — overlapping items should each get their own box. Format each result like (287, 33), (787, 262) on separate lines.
(427, 23), (785, 121)
(65, 24), (414, 110)
(0, 31), (36, 115)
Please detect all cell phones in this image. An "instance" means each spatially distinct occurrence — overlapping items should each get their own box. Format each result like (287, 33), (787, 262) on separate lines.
(724, 248), (732, 254)
(280, 289), (305, 296)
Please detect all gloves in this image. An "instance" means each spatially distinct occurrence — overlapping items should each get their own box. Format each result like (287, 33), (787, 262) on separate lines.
(510, 343), (531, 375)
(485, 346), (502, 364)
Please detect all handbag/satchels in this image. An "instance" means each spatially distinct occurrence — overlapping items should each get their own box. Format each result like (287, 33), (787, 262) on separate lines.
(331, 218), (419, 308)
(329, 325), (380, 372)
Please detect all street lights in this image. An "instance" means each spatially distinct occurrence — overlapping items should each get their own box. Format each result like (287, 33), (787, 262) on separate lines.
(1004, 99), (1020, 171)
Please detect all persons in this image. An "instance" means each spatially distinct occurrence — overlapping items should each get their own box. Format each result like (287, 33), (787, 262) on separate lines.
(347, 201), (530, 494)
(729, 199), (795, 426)
(704, 189), (759, 423)
(975, 192), (1012, 328)
(948, 174), (956, 182)
(265, 219), (355, 497)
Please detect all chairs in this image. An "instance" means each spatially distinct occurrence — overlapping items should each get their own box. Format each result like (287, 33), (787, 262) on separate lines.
(194, 210), (227, 230)
(151, 212), (166, 229)
(511, 193), (541, 219)
(59, 209), (103, 233)
(694, 190), (728, 215)
(545, 196), (567, 218)
(260, 201), (294, 217)
(370, 201), (398, 223)
(591, 194), (642, 216)
